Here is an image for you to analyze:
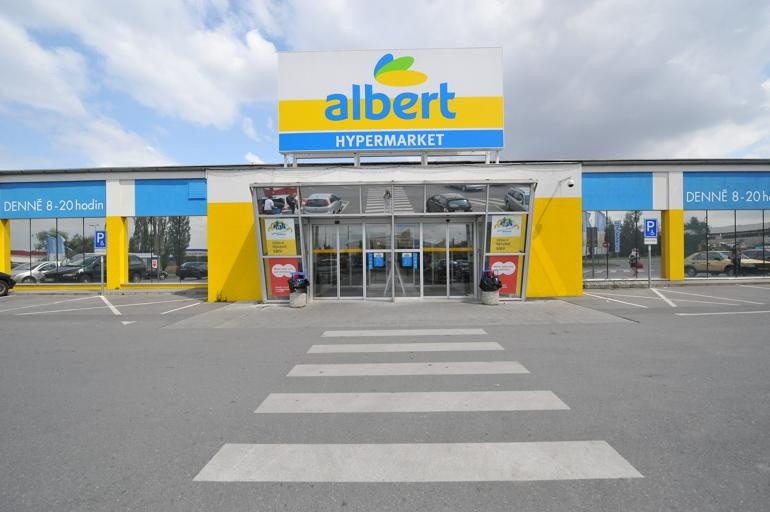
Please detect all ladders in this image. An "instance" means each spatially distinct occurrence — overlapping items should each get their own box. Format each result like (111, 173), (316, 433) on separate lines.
(383, 260), (407, 297)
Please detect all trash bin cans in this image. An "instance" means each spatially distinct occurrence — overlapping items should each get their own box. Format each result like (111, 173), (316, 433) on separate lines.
(289, 272), (308, 308)
(479, 270), (502, 306)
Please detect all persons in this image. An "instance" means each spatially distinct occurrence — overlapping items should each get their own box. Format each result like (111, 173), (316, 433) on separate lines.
(384, 189), (392, 212)
(263, 196), (278, 213)
(630, 248), (642, 274)
(732, 243), (743, 275)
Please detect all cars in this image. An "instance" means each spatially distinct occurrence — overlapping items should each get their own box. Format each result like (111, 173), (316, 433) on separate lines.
(426, 194), (473, 212)
(303, 193), (342, 213)
(12, 261), (63, 283)
(685, 251), (770, 276)
(446, 185), (486, 192)
(175, 262), (207, 280)
(425, 259), (472, 282)
(145, 268), (168, 279)
(739, 249), (770, 261)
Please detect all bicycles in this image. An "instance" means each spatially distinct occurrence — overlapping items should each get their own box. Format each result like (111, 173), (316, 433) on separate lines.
(627, 257), (645, 277)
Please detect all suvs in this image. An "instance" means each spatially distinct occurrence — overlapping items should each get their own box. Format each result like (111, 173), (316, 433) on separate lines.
(504, 185), (530, 211)
(41, 252), (145, 283)
(259, 194), (300, 214)
(317, 259), (337, 283)
(0, 272), (16, 296)
